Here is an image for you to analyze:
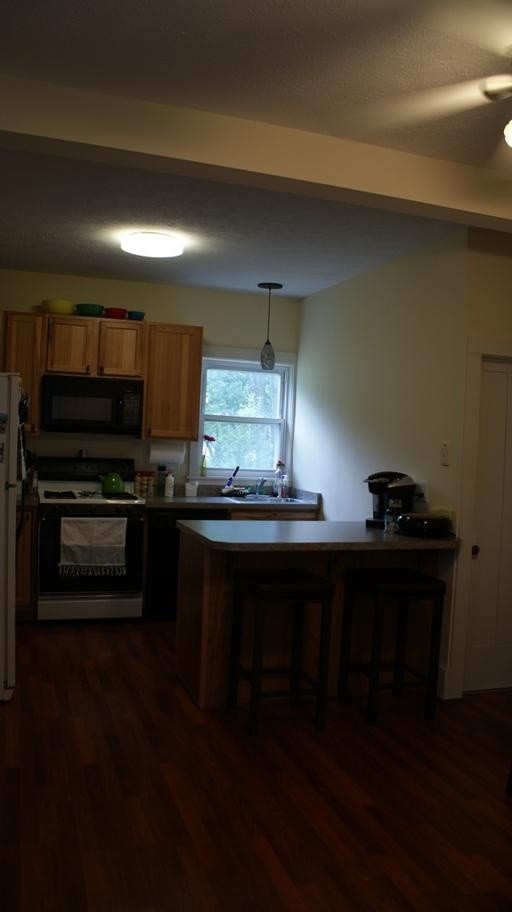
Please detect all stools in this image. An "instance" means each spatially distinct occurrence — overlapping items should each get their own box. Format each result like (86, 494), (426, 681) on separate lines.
(337, 566), (447, 726)
(224, 567), (336, 738)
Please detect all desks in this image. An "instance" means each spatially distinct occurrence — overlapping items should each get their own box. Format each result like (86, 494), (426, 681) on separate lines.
(175, 518), (461, 712)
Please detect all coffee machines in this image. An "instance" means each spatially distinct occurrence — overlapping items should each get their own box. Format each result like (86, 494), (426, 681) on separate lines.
(363, 470), (417, 529)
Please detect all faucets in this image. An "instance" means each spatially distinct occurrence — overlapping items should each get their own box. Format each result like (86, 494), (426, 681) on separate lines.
(255, 477), (268, 494)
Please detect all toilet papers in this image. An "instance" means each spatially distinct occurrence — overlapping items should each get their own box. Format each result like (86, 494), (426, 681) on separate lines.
(147, 442), (187, 466)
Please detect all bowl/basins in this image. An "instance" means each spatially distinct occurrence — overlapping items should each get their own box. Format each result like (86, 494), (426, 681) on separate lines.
(127, 311), (145, 321)
(104, 307), (127, 320)
(42, 298), (75, 315)
(77, 303), (103, 317)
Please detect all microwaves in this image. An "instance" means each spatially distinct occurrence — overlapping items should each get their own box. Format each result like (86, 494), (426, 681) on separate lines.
(41, 373), (144, 438)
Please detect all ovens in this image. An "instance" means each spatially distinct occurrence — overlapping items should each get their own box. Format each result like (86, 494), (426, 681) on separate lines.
(38, 507), (150, 597)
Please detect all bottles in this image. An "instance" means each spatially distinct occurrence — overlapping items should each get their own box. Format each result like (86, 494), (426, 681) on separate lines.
(163, 469), (176, 498)
(383, 508), (395, 534)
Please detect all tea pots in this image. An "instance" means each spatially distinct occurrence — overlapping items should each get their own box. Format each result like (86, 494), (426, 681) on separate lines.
(98, 471), (125, 494)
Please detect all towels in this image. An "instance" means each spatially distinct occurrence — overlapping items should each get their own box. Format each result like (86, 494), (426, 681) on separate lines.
(58, 517), (128, 577)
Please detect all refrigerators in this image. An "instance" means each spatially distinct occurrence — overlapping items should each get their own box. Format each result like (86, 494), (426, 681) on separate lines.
(0, 372), (22, 704)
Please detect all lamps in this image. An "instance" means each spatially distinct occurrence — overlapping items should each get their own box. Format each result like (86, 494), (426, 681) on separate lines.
(120, 232), (184, 258)
(257, 282), (282, 370)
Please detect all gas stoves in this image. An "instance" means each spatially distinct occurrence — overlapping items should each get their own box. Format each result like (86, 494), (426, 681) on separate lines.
(37, 489), (141, 502)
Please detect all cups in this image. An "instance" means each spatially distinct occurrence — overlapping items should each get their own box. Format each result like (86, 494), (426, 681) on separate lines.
(184, 481), (199, 497)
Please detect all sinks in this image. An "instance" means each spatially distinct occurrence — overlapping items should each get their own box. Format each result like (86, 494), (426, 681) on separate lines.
(224, 493), (304, 503)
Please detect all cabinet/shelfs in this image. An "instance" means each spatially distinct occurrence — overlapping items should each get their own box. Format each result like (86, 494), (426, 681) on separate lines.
(0, 310), (47, 434)
(43, 312), (145, 381)
(147, 323), (204, 442)
